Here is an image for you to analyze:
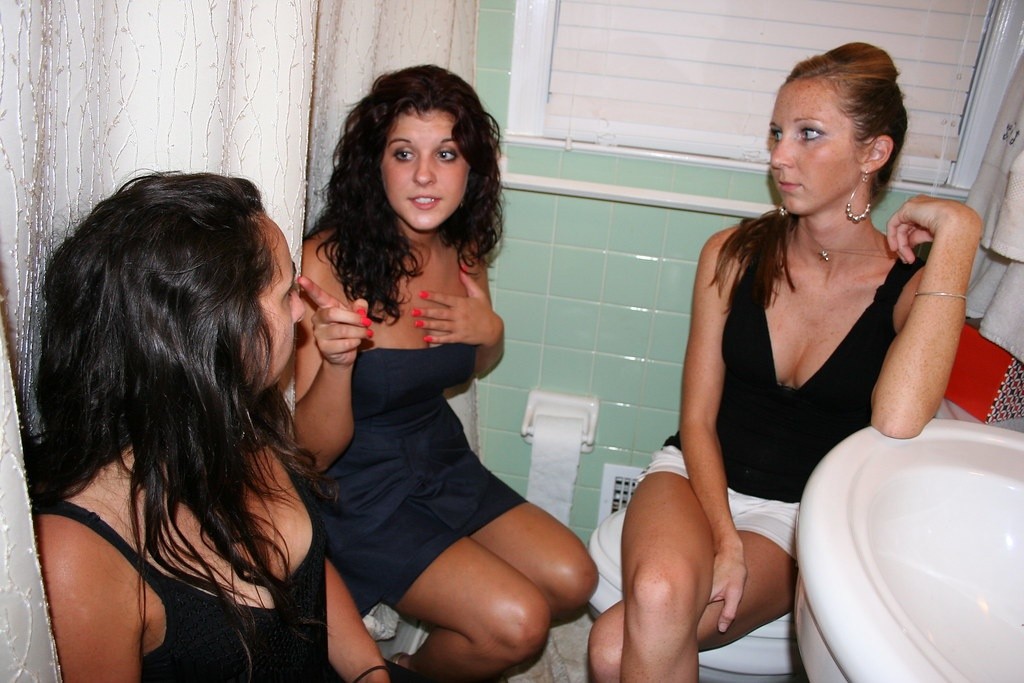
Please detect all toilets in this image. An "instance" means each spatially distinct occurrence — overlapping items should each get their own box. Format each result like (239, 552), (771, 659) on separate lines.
(583, 316), (1024, 683)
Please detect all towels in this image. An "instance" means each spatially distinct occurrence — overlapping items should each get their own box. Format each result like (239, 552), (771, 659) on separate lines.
(963, 51), (1024, 366)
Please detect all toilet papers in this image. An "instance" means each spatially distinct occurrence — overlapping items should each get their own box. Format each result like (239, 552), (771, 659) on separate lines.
(524, 413), (585, 530)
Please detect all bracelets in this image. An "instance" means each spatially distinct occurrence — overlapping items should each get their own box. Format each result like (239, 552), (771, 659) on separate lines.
(915, 292), (967, 300)
(354, 665), (388, 682)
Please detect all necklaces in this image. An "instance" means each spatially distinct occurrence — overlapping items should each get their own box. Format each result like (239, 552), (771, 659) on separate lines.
(800, 223), (899, 263)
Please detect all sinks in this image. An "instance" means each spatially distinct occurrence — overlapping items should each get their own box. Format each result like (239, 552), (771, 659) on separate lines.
(792, 415), (1023, 683)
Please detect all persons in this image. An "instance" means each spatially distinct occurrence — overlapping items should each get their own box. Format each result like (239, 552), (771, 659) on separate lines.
(266, 66), (599, 683)
(586, 41), (984, 683)
(32, 171), (393, 682)
